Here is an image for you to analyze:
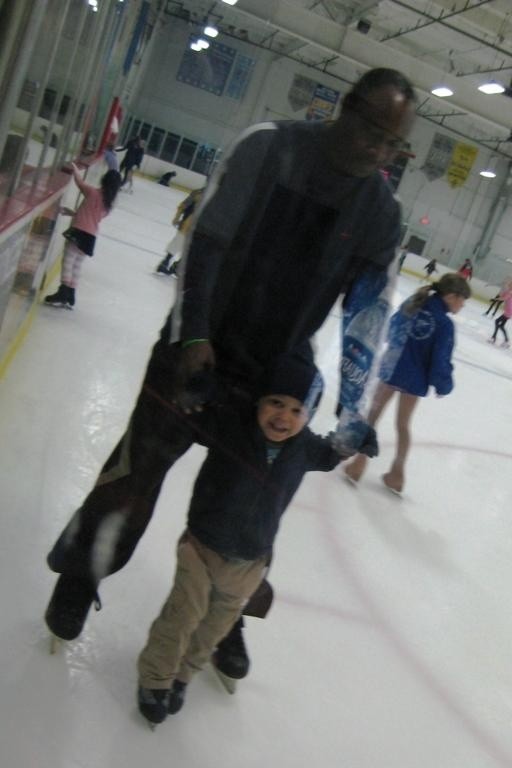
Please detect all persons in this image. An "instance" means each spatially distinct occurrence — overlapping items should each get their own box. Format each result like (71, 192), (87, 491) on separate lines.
(397, 244), (408, 272)
(39, 65), (428, 687)
(161, 171), (177, 186)
(14, 205), (56, 302)
(423, 259), (436, 278)
(44, 162), (120, 307)
(159, 188), (206, 276)
(460, 259), (472, 278)
(105, 135), (144, 192)
(343, 273), (471, 499)
(485, 280), (512, 348)
(136, 354), (366, 727)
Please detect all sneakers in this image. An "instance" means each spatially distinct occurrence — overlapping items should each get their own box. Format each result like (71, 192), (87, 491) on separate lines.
(167, 678), (187, 714)
(138, 687), (170, 722)
(45, 284), (70, 304)
(44, 574), (101, 641)
(66, 287), (74, 304)
(210, 614), (250, 679)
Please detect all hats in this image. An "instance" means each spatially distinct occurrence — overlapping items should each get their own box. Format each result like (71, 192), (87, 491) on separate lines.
(255, 350), (324, 418)
